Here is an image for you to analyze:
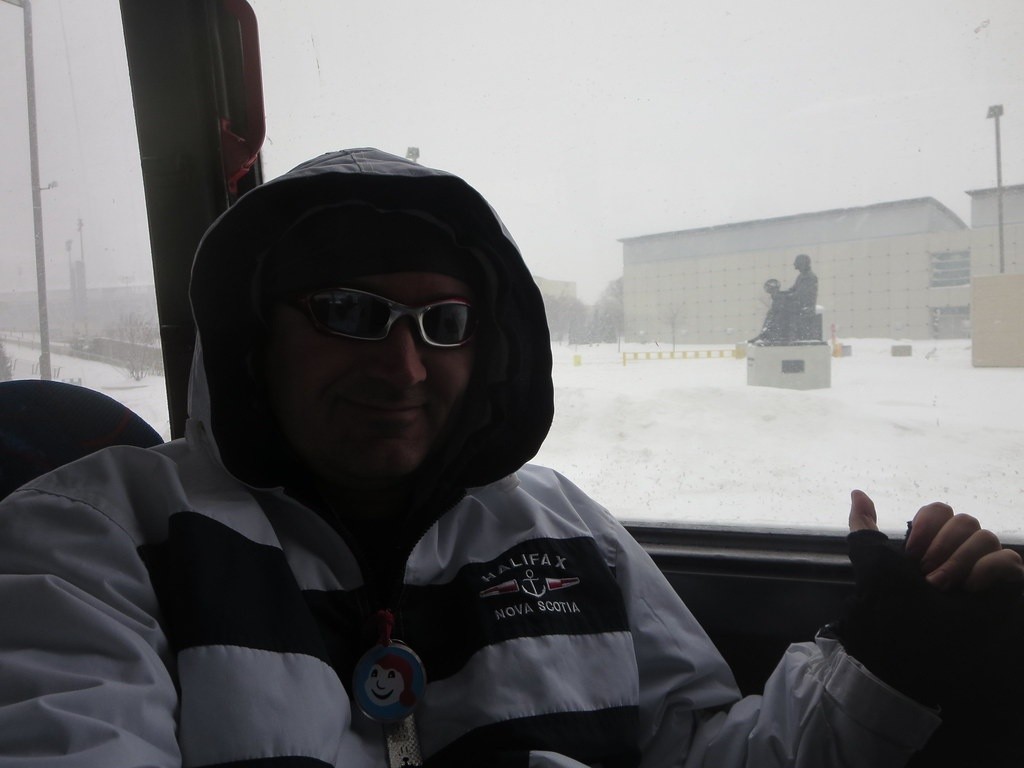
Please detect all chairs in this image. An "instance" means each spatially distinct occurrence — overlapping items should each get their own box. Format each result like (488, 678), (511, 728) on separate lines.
(1, 380), (167, 503)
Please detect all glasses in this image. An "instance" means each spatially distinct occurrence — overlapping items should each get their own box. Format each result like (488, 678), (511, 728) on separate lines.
(275, 281), (487, 348)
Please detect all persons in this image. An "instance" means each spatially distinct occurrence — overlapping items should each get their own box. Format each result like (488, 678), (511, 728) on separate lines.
(0, 148), (1024, 768)
(748, 254), (826, 345)
